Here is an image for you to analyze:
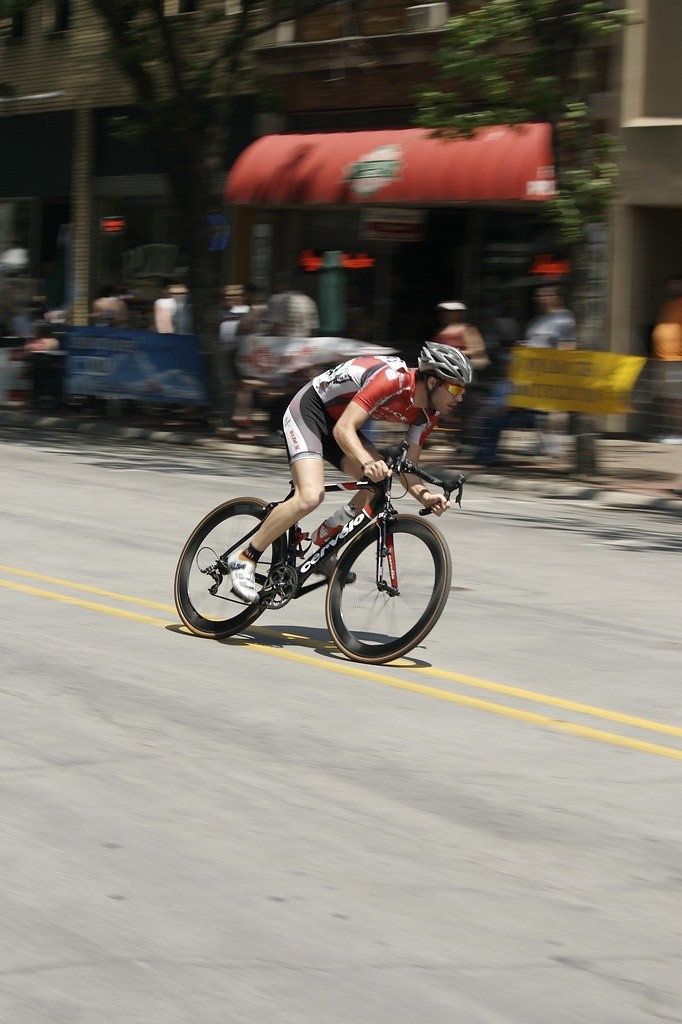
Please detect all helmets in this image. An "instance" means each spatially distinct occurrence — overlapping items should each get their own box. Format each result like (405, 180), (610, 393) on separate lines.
(418, 341), (473, 385)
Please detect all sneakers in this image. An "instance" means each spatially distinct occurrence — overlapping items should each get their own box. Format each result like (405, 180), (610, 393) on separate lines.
(317, 554), (355, 582)
(227, 550), (259, 603)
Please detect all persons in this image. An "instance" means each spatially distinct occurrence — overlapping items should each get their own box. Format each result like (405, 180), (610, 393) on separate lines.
(432, 297), (494, 466)
(648, 273), (682, 366)
(25, 275), (324, 415)
(220, 338), (473, 610)
(500, 282), (575, 453)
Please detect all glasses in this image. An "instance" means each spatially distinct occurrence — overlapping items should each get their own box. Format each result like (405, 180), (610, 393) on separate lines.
(447, 385), (466, 396)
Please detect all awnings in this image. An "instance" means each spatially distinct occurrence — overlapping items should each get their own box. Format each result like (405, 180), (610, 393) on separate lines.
(213, 125), (561, 209)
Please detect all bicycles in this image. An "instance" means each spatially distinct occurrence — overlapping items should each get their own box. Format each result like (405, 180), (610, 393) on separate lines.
(174, 441), (467, 664)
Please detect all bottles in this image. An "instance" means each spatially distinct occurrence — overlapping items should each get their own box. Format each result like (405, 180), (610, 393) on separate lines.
(311, 505), (357, 548)
